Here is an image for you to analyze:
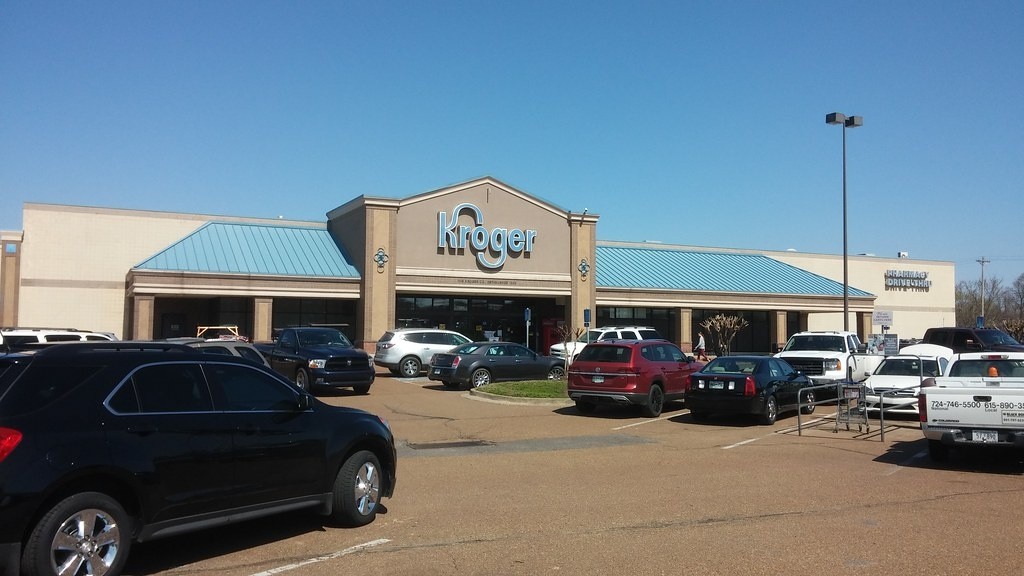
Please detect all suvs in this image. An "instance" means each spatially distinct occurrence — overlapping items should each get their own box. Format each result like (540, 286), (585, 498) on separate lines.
(549, 326), (661, 362)
(774, 329), (870, 400)
(566, 339), (703, 419)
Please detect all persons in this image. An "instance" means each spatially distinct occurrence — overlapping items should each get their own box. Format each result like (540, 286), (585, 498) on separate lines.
(867, 337), (884, 356)
(694, 331), (711, 362)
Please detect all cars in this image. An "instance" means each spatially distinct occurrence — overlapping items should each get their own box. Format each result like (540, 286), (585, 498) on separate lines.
(923, 327), (1024, 354)
(373, 329), (472, 377)
(857, 344), (953, 419)
(682, 353), (817, 424)
(426, 339), (565, 386)
(1, 324), (398, 576)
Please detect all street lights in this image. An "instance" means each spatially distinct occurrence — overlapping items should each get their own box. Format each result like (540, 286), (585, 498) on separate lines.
(825, 110), (864, 333)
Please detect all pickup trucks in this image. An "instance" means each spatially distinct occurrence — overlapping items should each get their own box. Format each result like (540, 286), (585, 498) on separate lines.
(252, 327), (375, 395)
(920, 352), (1024, 460)
